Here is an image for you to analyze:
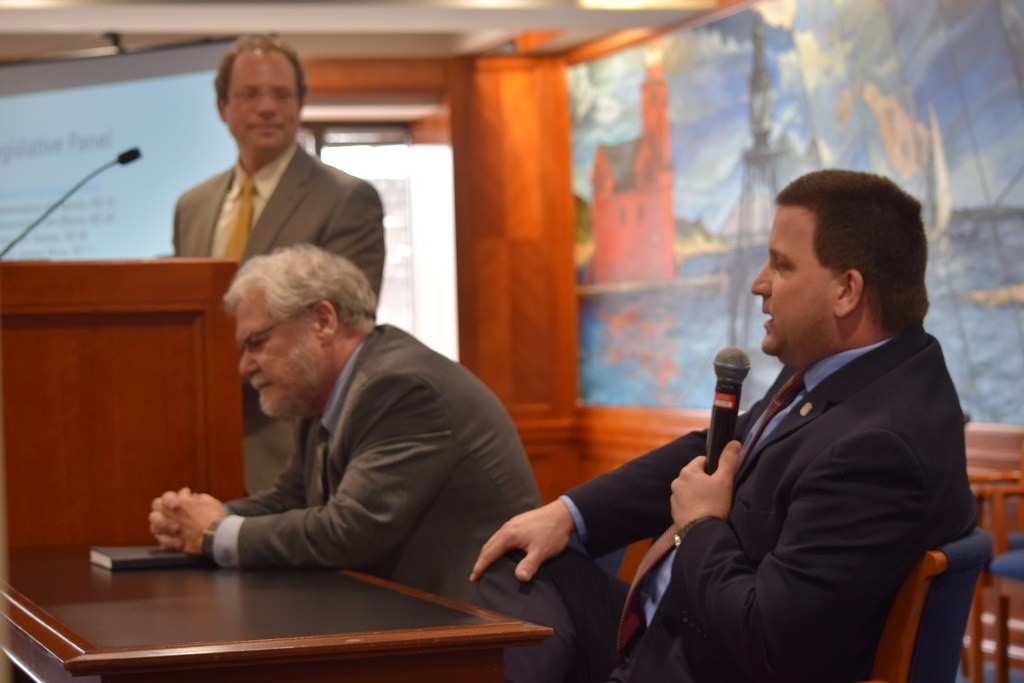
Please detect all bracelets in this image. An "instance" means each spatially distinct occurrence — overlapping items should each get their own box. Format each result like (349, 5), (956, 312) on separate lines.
(201, 516), (226, 557)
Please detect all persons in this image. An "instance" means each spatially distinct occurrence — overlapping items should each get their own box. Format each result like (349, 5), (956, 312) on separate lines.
(171, 37), (385, 497)
(470, 170), (979, 683)
(149, 244), (577, 683)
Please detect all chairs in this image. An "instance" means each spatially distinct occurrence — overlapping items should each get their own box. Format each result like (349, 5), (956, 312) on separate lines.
(868, 465), (1023, 683)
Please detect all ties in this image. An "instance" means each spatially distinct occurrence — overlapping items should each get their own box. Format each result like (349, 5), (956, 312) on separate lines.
(616, 371), (805, 651)
(219, 175), (257, 258)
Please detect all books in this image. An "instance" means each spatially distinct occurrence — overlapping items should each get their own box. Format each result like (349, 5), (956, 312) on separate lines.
(89, 546), (201, 570)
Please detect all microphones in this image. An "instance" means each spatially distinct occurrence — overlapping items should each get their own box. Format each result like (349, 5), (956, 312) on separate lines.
(705, 348), (751, 476)
(0, 148), (142, 260)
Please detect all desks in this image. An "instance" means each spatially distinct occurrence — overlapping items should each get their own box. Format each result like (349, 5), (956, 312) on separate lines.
(0, 541), (555, 683)
